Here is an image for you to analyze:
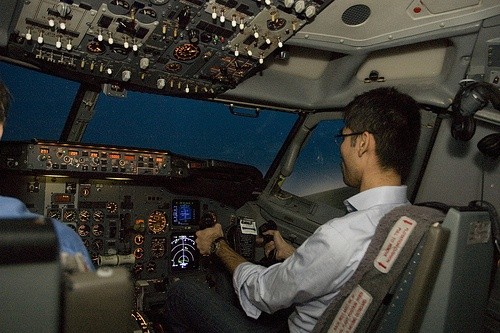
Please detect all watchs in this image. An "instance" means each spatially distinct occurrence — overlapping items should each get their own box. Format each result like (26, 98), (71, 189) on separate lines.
(209, 237), (225, 257)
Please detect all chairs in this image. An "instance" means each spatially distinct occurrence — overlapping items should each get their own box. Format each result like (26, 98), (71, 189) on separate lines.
(311, 206), (498, 332)
(1, 208), (139, 332)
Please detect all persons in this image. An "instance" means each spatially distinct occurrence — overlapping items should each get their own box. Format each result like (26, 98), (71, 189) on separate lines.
(0, 76), (96, 274)
(168, 86), (422, 333)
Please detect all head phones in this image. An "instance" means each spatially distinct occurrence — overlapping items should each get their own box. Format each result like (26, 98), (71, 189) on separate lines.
(452, 81), (500, 157)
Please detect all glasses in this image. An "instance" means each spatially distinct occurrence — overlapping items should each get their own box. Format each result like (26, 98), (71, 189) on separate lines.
(334, 130), (379, 146)
